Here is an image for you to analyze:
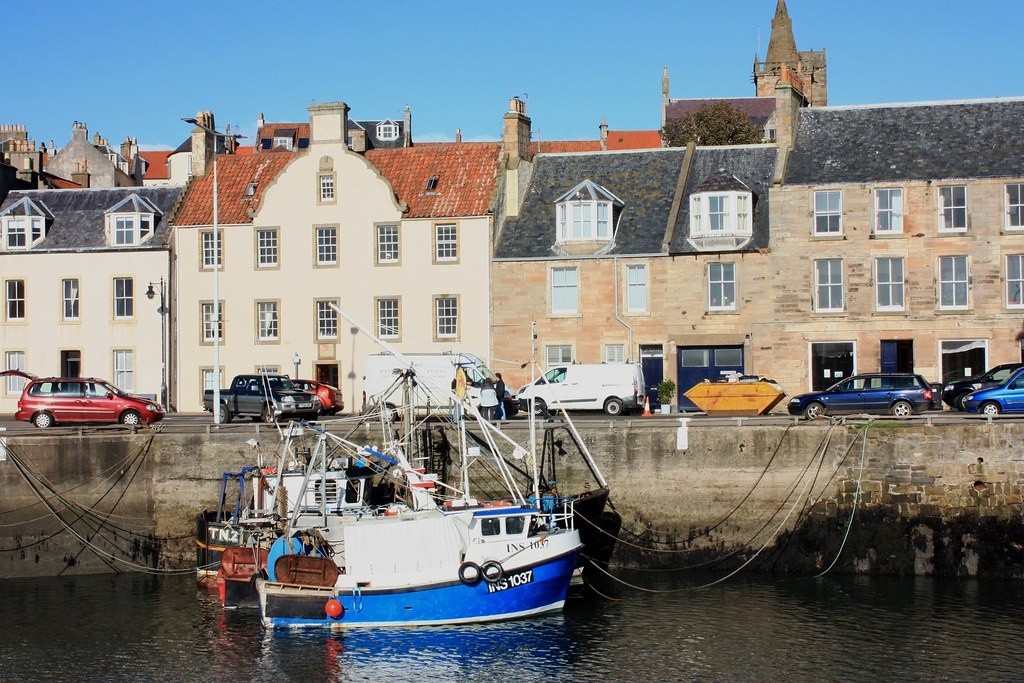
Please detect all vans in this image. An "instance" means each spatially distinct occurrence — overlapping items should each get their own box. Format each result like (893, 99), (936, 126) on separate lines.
(518, 364), (646, 416)
(361, 351), (520, 421)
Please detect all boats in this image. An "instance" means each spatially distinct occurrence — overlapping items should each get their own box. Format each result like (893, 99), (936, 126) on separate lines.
(255, 421), (581, 630)
(193, 321), (613, 610)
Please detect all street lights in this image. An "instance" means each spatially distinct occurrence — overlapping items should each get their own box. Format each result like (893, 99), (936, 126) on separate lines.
(181, 116), (249, 430)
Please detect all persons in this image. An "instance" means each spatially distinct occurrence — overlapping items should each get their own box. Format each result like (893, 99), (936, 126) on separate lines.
(480, 378), (499, 420)
(495, 373), (506, 420)
(451, 370), (474, 422)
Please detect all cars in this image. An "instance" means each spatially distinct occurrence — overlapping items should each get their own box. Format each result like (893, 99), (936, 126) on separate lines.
(963, 367), (1024, 415)
(940, 362), (1024, 411)
(786, 372), (935, 420)
(14, 376), (165, 427)
(287, 379), (344, 416)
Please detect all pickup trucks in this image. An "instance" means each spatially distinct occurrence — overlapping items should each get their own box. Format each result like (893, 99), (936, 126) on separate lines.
(202, 373), (322, 429)
